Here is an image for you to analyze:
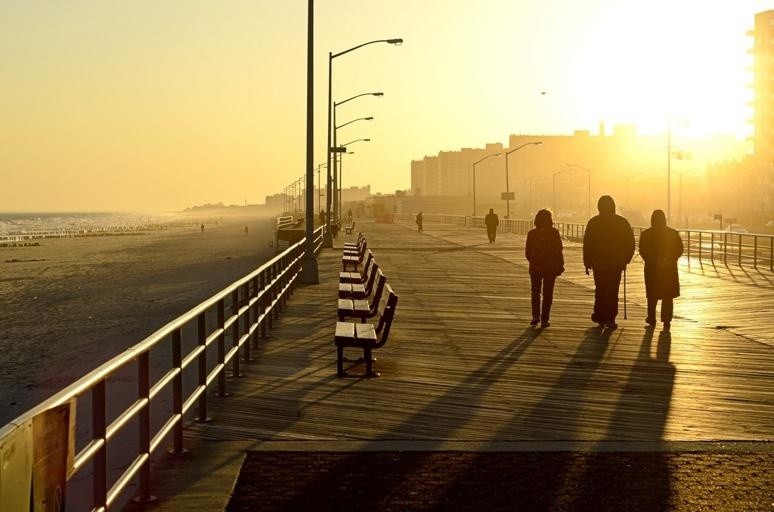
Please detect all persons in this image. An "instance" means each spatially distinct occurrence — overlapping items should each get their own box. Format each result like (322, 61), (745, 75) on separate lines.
(583, 195), (635, 330)
(319, 209), (325, 225)
(485, 208), (499, 244)
(201, 224), (204, 232)
(244, 226), (248, 235)
(639, 209), (683, 328)
(348, 208), (352, 223)
(525, 209), (564, 327)
(416, 212), (423, 232)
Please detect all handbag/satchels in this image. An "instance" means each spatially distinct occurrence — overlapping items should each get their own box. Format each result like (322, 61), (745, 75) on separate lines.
(550, 252), (565, 277)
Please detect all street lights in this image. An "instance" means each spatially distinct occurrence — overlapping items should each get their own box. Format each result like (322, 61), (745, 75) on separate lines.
(280, 150), (357, 226)
(330, 116), (371, 237)
(506, 141), (544, 219)
(331, 90), (384, 238)
(566, 162), (592, 221)
(552, 167), (576, 218)
(612, 169), (644, 208)
(332, 138), (370, 233)
(322, 36), (404, 249)
(472, 153), (502, 216)
(663, 166), (699, 226)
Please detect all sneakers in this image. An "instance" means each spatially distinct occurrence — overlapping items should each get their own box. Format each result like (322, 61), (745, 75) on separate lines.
(541, 323), (550, 328)
(530, 318), (539, 326)
(645, 317), (656, 325)
(599, 320), (618, 337)
(663, 320), (670, 331)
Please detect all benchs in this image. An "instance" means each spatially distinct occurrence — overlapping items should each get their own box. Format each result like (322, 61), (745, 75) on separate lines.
(334, 217), (400, 381)
(290, 216), (304, 230)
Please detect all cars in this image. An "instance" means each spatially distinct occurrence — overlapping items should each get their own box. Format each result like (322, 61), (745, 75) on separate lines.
(727, 224), (748, 235)
